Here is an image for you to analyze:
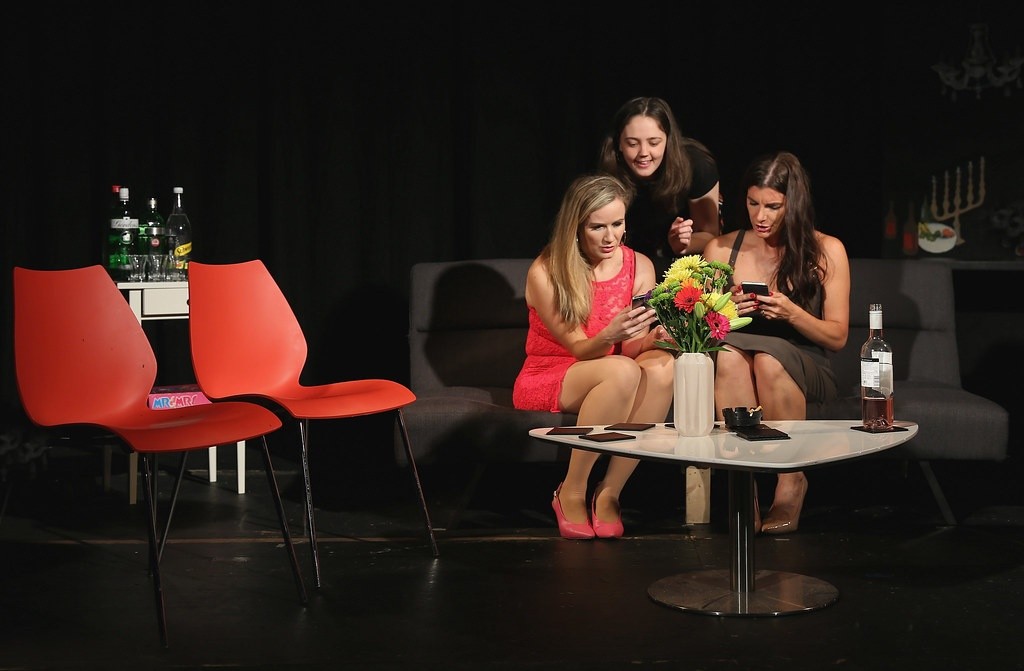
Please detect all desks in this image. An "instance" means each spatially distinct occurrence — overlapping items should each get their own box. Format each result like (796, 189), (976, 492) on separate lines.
(100, 282), (249, 503)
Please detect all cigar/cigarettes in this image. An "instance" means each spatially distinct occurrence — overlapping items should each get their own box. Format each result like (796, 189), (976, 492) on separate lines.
(749, 406), (761, 416)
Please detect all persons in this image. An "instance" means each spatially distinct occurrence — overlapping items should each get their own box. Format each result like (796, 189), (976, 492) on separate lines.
(514, 95), (851, 538)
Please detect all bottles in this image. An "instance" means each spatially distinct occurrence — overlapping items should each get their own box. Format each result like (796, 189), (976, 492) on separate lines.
(140, 197), (166, 253)
(166, 188), (189, 281)
(109, 185), (139, 273)
(860, 303), (895, 432)
(145, 224), (165, 255)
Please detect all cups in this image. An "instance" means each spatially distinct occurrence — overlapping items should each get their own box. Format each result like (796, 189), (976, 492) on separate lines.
(167, 252), (190, 283)
(147, 254), (168, 281)
(125, 255), (146, 282)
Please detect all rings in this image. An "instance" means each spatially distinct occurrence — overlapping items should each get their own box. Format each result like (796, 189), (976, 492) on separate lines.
(762, 310), (764, 316)
(690, 229), (693, 234)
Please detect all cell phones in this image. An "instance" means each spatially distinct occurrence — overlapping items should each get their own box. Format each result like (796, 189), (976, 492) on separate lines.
(741, 282), (769, 307)
(631, 295), (653, 318)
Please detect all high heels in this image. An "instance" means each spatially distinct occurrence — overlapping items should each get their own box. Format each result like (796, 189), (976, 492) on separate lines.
(754, 476), (808, 535)
(552, 482), (624, 539)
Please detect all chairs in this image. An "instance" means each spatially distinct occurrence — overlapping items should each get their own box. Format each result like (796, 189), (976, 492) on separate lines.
(160, 261), (439, 589)
(13, 262), (311, 651)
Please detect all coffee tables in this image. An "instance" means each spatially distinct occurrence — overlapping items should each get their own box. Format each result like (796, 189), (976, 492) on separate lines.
(521, 417), (918, 619)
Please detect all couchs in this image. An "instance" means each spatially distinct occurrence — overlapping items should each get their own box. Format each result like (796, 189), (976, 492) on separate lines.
(395, 260), (1007, 525)
